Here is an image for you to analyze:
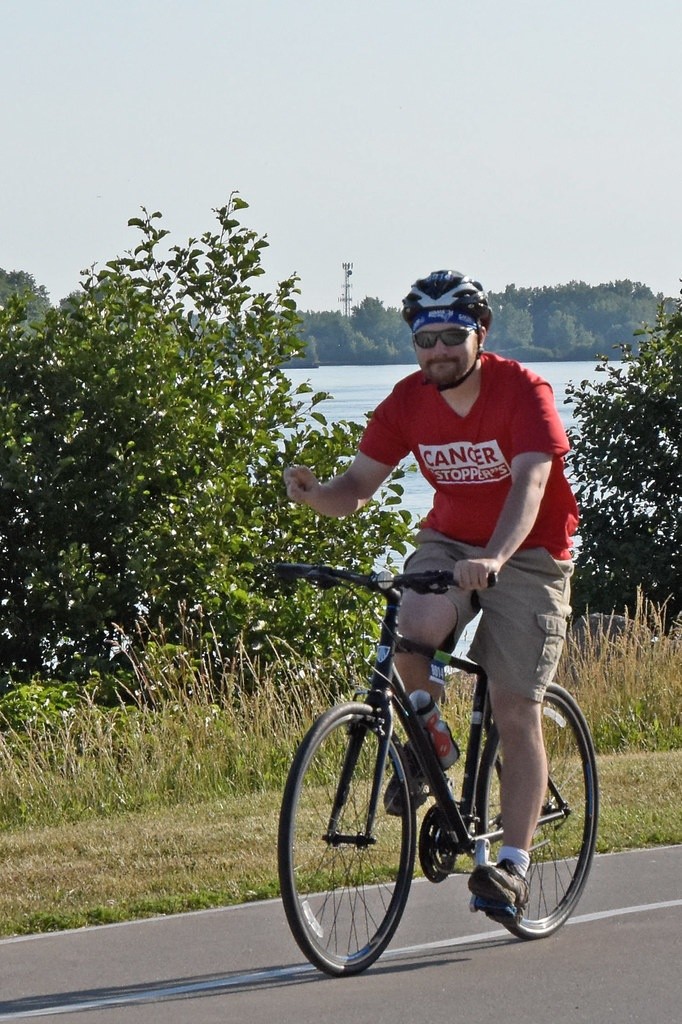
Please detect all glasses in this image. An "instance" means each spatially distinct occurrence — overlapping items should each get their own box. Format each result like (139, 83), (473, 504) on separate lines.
(410, 328), (474, 348)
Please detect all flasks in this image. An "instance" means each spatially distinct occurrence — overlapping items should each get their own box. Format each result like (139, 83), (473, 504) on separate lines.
(409, 690), (459, 768)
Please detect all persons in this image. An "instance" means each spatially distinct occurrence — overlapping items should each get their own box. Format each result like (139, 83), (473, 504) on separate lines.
(282, 269), (580, 927)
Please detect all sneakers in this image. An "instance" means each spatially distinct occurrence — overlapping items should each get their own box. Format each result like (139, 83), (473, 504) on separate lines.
(383, 743), (430, 816)
(468, 859), (530, 923)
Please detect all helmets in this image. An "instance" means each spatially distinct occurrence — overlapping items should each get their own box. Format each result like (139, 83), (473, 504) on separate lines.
(402, 270), (493, 331)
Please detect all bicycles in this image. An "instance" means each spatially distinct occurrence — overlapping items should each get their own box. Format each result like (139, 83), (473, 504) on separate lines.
(274, 562), (599, 978)
